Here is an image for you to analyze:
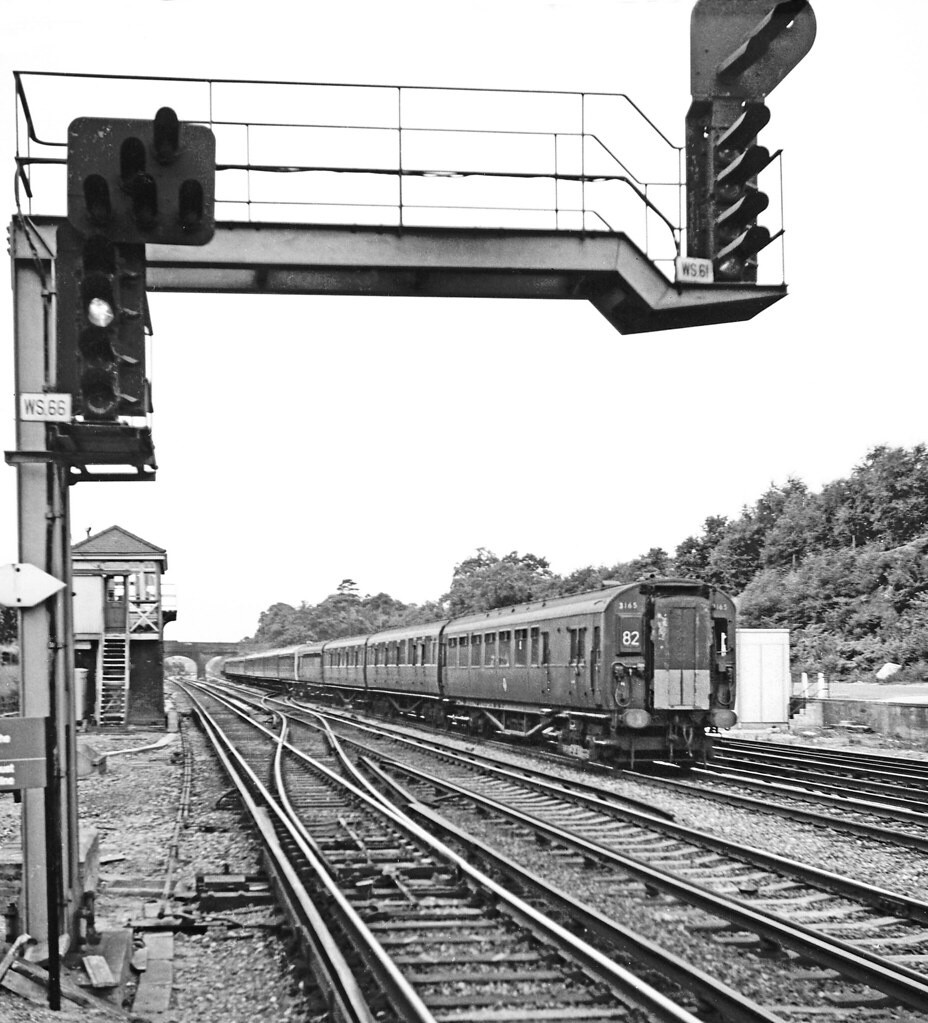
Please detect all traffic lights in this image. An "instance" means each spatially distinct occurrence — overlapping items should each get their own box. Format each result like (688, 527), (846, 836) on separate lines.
(687, 0), (816, 283)
(67, 107), (214, 246)
(56, 222), (148, 420)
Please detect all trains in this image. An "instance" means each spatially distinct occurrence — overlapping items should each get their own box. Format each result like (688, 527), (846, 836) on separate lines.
(221, 576), (739, 768)
